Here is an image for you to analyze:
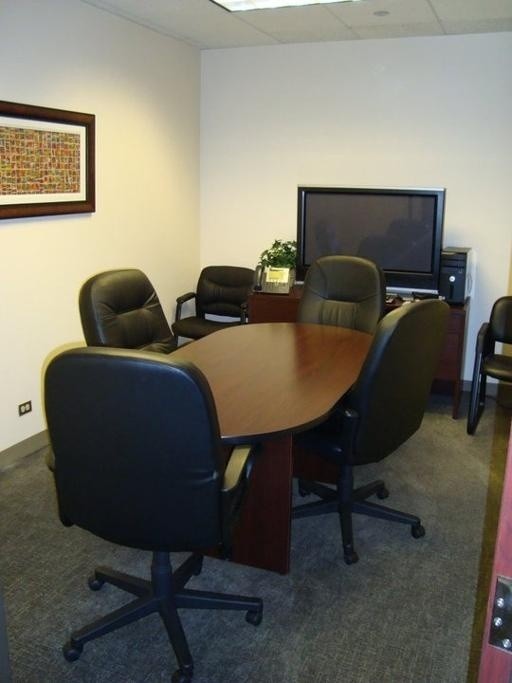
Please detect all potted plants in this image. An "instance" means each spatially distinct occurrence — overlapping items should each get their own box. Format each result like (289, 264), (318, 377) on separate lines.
(256, 238), (298, 288)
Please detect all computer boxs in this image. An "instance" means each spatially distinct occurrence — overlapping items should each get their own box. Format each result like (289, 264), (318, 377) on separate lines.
(438, 247), (471, 307)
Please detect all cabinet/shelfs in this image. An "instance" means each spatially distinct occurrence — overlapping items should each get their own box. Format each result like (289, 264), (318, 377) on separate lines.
(246, 285), (470, 420)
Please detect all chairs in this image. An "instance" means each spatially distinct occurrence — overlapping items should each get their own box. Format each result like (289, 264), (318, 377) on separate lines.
(294, 255), (386, 336)
(77, 268), (193, 354)
(467, 296), (512, 434)
(287, 299), (451, 565)
(43, 346), (263, 683)
(170, 266), (257, 347)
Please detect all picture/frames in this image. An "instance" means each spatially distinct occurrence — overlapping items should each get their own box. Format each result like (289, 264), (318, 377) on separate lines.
(0, 100), (95, 219)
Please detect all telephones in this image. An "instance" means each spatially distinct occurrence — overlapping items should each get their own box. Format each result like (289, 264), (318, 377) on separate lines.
(252, 265), (295, 294)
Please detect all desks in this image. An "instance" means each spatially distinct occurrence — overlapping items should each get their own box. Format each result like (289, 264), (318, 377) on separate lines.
(163, 321), (370, 577)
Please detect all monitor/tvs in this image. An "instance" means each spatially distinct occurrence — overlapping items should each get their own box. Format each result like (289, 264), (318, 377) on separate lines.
(295, 183), (446, 298)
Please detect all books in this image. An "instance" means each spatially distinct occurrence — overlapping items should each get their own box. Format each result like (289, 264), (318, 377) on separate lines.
(386, 294), (446, 306)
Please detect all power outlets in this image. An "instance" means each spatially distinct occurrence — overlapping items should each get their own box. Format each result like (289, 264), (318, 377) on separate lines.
(18, 401), (31, 416)
